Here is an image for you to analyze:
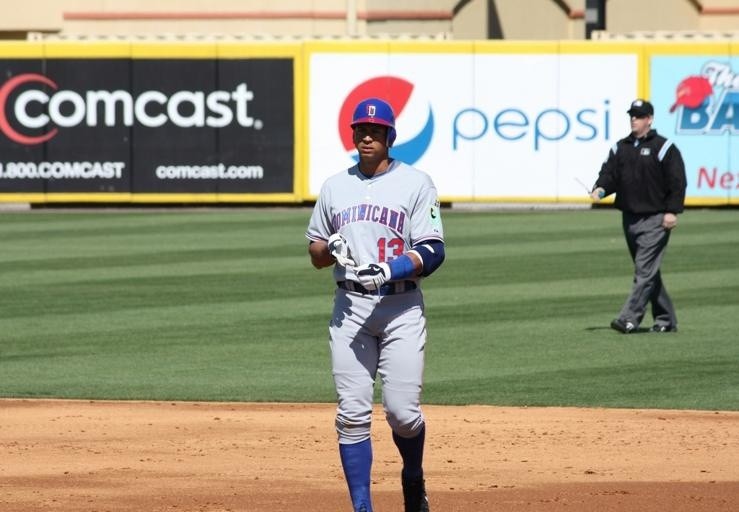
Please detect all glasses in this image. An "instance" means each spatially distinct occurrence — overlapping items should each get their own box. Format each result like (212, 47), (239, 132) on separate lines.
(630, 111), (646, 118)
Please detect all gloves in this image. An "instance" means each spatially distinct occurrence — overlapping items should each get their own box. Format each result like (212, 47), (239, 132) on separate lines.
(353, 262), (391, 291)
(327, 233), (355, 267)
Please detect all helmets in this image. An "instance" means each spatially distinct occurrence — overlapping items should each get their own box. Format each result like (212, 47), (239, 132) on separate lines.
(351, 98), (396, 147)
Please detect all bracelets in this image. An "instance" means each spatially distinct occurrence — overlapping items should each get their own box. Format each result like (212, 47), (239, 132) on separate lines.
(386, 255), (413, 281)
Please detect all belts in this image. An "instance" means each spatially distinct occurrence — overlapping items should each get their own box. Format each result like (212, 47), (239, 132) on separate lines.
(337, 281), (416, 295)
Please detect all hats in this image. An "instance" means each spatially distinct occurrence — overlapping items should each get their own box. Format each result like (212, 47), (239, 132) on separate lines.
(670, 76), (711, 112)
(628, 100), (653, 115)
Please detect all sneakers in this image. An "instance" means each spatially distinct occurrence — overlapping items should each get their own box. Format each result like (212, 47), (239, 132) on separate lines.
(611, 319), (636, 334)
(647, 325), (678, 332)
(402, 466), (429, 512)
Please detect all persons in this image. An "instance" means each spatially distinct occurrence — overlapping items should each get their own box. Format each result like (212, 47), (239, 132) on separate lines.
(304, 99), (445, 512)
(591, 99), (687, 334)
